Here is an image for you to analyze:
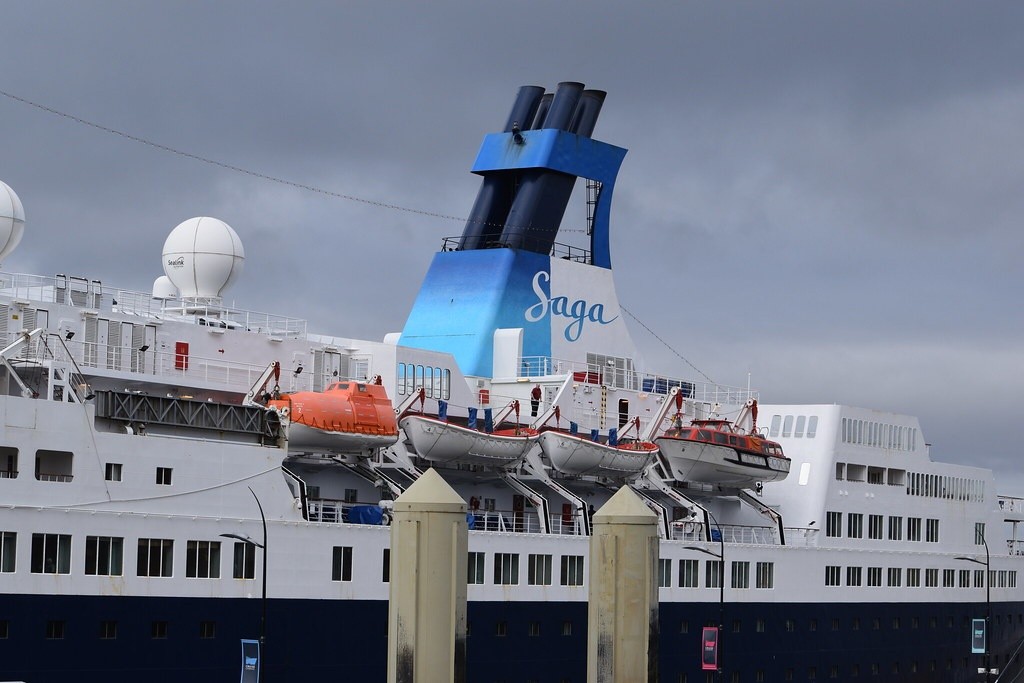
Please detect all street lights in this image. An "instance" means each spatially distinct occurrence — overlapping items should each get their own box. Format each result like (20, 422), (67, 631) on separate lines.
(954, 527), (992, 682)
(218, 484), (266, 682)
(683, 508), (725, 683)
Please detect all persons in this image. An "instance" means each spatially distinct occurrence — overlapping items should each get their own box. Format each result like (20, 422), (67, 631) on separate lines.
(466, 508), (475, 530)
(384, 510), (393, 526)
(113, 299), (117, 305)
(587, 505), (597, 535)
(470, 496), (481, 510)
(531, 383), (542, 417)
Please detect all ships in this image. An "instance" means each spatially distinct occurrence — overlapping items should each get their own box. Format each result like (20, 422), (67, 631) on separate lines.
(1, 0), (1024, 683)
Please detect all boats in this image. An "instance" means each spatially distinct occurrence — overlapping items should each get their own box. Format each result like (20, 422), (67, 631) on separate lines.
(537, 410), (660, 478)
(650, 419), (793, 489)
(242, 360), (402, 453)
(398, 381), (543, 471)
